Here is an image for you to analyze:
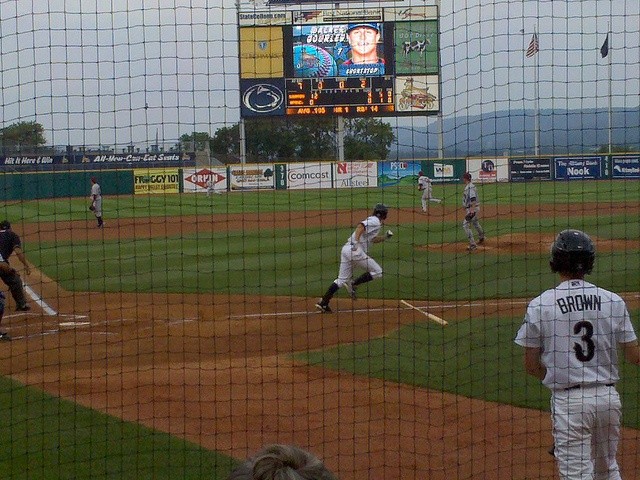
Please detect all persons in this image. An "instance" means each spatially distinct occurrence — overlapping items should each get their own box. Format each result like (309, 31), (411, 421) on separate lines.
(342, 24), (381, 64)
(0, 220), (31, 312)
(512, 230), (640, 480)
(314, 203), (393, 314)
(462, 172), (486, 251)
(417, 171), (442, 213)
(88, 176), (105, 228)
(226, 443), (338, 480)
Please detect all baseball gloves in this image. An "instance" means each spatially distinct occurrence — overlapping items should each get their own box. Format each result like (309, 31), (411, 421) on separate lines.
(89, 206), (95, 211)
(464, 211), (476, 221)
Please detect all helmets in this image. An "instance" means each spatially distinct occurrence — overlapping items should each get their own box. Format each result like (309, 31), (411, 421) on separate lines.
(0, 220), (10, 230)
(373, 204), (388, 217)
(551, 229), (595, 270)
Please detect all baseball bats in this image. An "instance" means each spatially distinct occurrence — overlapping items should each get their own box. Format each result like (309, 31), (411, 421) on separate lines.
(400, 299), (448, 326)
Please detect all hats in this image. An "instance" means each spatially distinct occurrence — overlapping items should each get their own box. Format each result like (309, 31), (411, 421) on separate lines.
(346, 23), (379, 32)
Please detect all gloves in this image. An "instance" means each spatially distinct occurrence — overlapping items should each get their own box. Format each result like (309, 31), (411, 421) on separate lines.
(386, 230), (393, 238)
(350, 240), (359, 251)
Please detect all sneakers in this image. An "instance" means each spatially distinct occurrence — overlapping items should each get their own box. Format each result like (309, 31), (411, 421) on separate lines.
(16, 304), (31, 311)
(342, 280), (358, 300)
(477, 237), (488, 245)
(467, 243), (477, 251)
(315, 297), (332, 313)
